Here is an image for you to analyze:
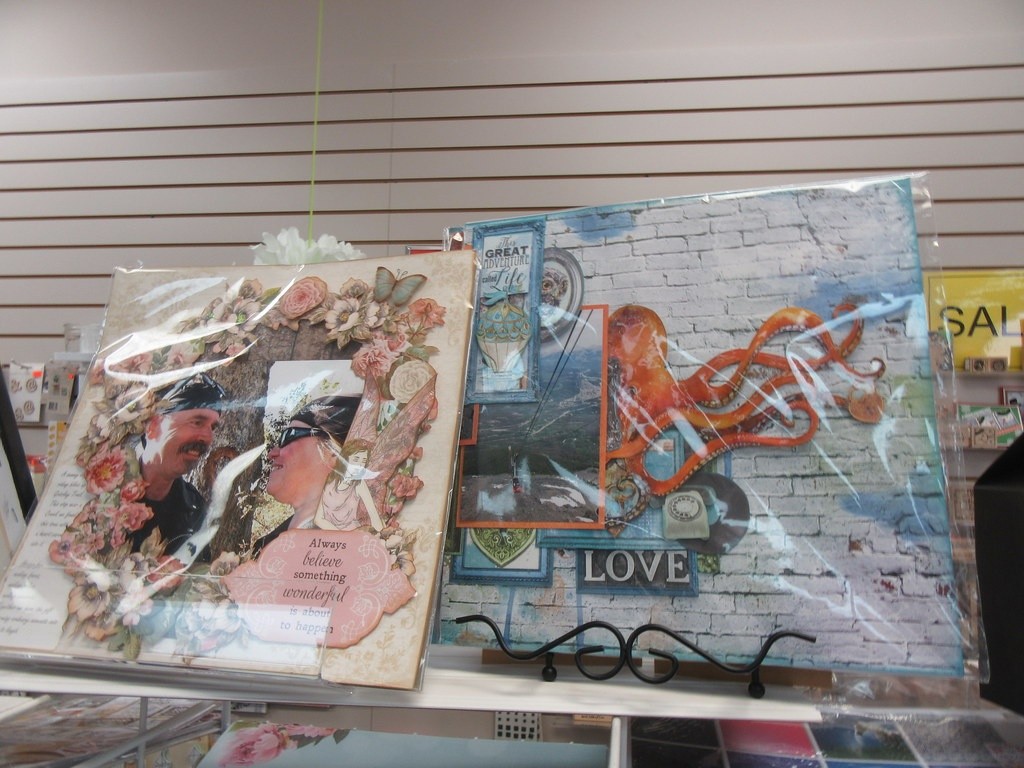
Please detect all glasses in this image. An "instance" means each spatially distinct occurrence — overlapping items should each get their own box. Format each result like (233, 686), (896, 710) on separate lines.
(279, 425), (329, 448)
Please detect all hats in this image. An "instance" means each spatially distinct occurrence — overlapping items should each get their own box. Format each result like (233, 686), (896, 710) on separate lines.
(289, 393), (365, 444)
(151, 370), (223, 416)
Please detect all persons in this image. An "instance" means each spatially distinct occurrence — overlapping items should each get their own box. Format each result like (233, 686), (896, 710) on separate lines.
(128, 372), (225, 564)
(253, 394), (361, 564)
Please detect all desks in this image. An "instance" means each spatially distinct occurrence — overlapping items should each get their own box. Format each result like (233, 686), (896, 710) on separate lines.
(0, 644), (823, 768)
